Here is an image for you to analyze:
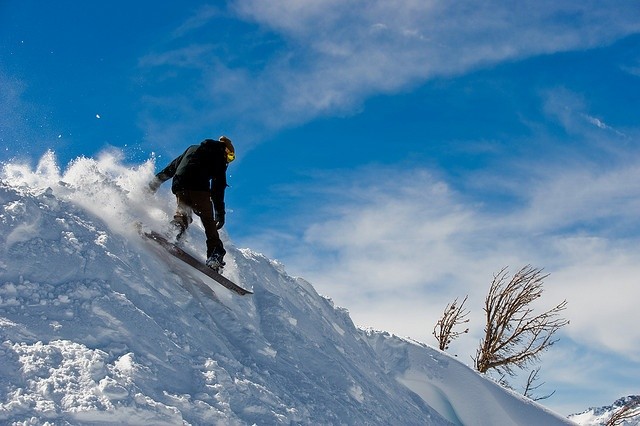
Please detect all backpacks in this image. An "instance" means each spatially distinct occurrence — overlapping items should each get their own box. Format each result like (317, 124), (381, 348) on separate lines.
(187, 139), (227, 178)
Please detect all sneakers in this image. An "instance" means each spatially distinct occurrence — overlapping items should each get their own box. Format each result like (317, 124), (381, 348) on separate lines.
(205, 254), (223, 275)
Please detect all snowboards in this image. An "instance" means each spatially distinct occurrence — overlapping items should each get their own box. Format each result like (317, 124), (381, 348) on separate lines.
(139, 225), (253, 296)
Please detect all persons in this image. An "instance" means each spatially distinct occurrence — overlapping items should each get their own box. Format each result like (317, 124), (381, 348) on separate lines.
(147, 136), (235, 273)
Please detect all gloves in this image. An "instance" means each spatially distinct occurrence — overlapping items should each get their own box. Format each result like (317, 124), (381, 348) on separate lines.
(214, 211), (225, 230)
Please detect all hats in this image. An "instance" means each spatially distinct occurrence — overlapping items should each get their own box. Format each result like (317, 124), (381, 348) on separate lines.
(219, 136), (235, 163)
